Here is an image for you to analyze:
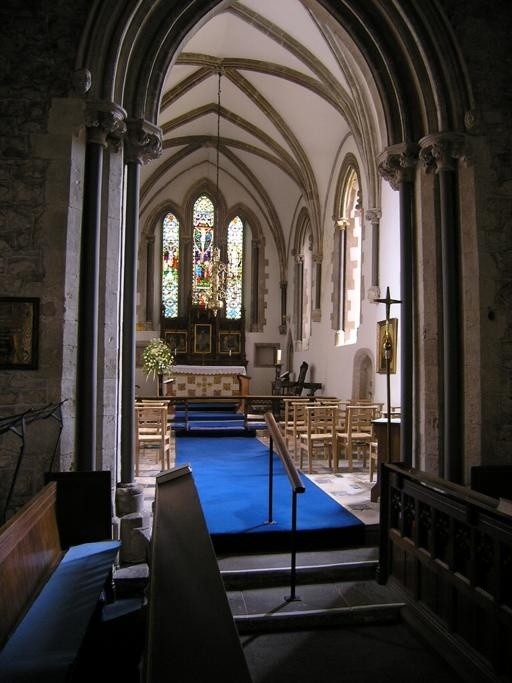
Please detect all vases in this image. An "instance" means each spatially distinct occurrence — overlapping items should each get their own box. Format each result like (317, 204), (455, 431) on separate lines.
(157, 373), (164, 395)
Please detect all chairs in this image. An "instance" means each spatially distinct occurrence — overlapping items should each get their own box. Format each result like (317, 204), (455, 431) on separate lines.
(279, 398), (402, 483)
(135, 395), (176, 477)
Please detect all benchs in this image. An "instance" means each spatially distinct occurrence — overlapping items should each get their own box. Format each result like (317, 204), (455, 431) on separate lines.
(0, 481), (124, 679)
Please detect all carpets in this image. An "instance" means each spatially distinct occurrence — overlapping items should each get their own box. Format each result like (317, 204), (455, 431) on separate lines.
(171, 400), (365, 537)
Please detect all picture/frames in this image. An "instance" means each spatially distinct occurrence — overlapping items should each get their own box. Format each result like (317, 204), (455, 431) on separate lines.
(375, 317), (398, 374)
(164, 323), (241, 356)
(1, 296), (40, 370)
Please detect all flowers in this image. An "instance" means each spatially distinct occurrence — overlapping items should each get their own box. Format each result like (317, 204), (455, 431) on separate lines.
(141, 337), (175, 383)
